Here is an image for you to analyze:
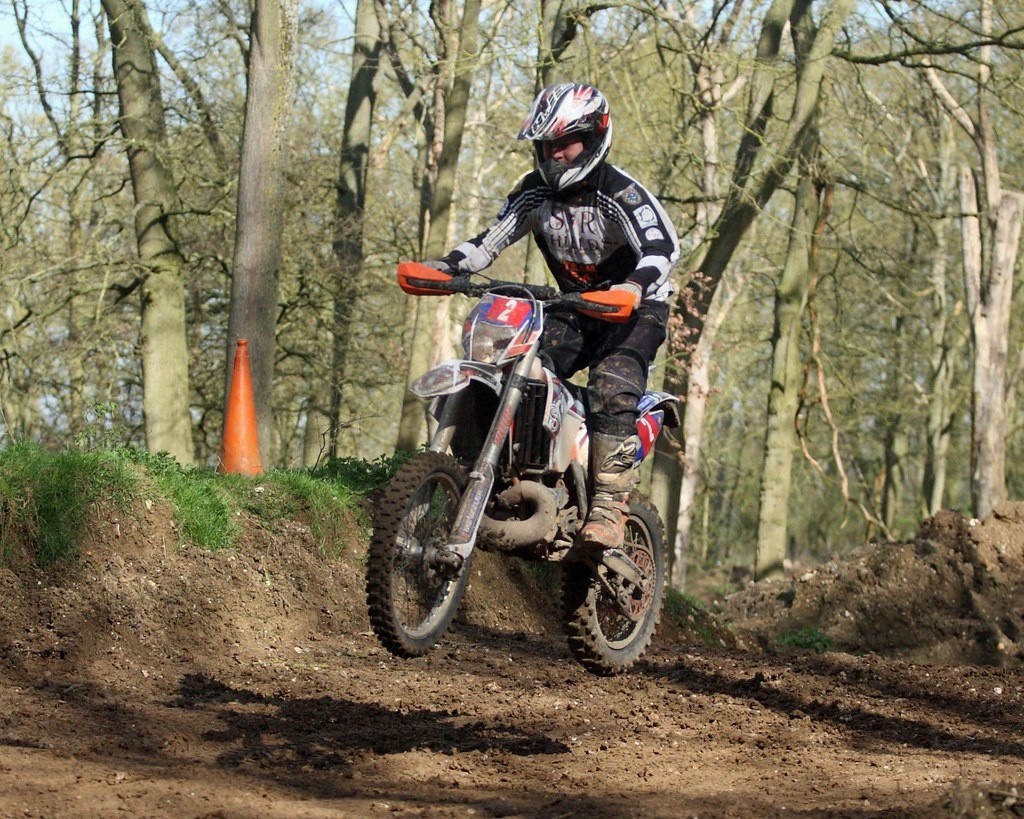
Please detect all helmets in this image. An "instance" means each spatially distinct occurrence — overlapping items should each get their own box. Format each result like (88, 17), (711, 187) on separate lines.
(519, 82), (614, 194)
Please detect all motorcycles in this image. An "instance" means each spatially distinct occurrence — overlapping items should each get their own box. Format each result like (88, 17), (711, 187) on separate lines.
(364, 264), (669, 678)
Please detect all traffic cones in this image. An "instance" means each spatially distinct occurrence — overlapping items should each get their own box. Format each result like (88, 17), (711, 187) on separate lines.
(217, 335), (264, 481)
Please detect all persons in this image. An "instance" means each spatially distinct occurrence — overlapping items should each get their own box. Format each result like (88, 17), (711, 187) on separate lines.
(418, 82), (680, 552)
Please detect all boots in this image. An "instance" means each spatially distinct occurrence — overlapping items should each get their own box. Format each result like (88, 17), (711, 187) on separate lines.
(581, 431), (637, 550)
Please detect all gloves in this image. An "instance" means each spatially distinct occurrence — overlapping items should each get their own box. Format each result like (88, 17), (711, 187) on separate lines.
(419, 258), (450, 273)
(608, 282), (643, 310)
